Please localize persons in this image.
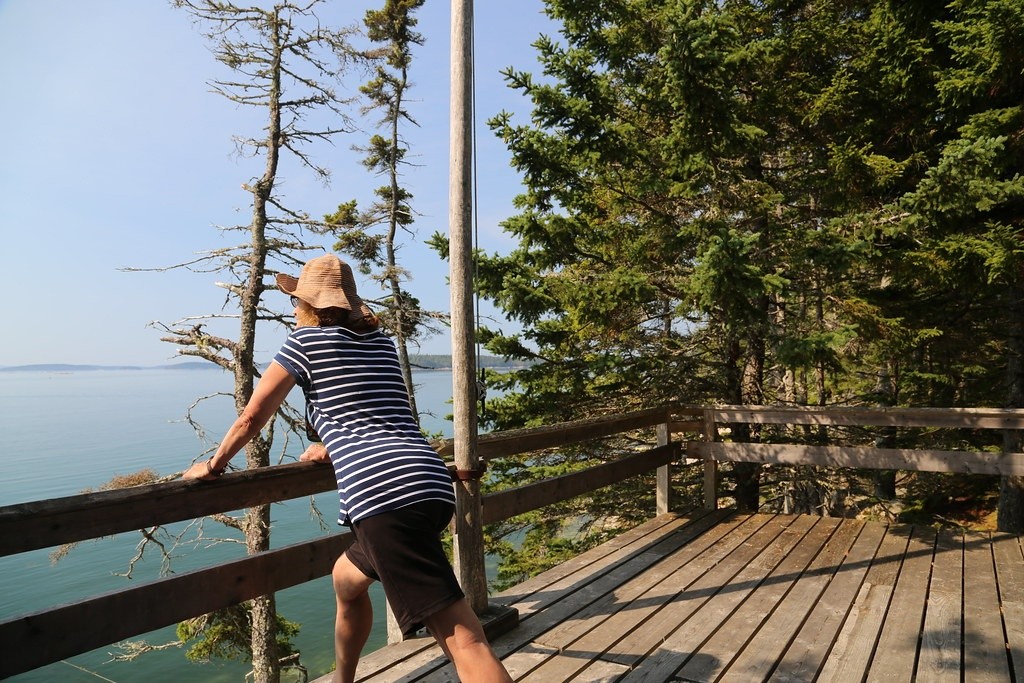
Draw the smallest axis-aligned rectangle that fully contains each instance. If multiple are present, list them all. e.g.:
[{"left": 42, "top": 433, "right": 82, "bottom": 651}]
[{"left": 178, "top": 254, "right": 515, "bottom": 683}]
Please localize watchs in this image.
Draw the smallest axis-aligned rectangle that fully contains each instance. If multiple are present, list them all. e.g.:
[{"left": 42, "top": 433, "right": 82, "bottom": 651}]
[{"left": 207, "top": 455, "right": 228, "bottom": 476}]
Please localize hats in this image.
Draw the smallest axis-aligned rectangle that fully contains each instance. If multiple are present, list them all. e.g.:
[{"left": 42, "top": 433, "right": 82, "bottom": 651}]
[{"left": 274, "top": 255, "right": 376, "bottom": 323}]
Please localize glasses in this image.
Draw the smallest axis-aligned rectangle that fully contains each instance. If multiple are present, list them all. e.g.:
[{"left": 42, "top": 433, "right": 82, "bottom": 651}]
[{"left": 288, "top": 293, "right": 301, "bottom": 307}]
